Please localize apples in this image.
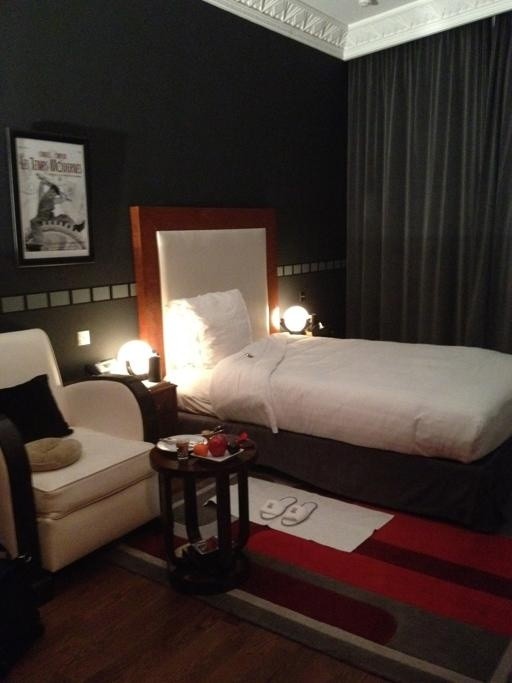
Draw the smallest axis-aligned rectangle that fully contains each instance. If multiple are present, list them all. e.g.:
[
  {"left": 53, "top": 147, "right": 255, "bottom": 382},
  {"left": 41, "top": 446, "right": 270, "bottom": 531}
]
[{"left": 208, "top": 437, "right": 227, "bottom": 457}]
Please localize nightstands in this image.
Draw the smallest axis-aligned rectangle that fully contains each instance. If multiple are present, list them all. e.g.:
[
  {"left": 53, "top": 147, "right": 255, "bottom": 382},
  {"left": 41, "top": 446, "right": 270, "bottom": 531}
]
[{"left": 134, "top": 378, "right": 178, "bottom": 442}]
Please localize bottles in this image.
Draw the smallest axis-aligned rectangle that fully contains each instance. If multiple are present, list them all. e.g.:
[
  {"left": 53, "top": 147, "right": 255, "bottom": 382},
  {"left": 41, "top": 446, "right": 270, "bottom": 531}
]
[{"left": 148, "top": 349, "right": 161, "bottom": 382}]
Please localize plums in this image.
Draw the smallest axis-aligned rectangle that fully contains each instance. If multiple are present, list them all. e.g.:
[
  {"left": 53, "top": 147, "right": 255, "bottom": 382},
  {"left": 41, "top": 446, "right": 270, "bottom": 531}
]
[{"left": 227, "top": 441, "right": 240, "bottom": 454}]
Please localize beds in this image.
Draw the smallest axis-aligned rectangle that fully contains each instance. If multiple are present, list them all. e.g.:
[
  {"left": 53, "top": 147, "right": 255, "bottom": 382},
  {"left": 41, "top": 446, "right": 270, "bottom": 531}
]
[{"left": 131, "top": 205, "right": 512, "bottom": 524}]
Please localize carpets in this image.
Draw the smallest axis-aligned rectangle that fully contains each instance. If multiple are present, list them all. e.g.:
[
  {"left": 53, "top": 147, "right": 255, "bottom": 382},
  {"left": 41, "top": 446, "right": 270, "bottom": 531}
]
[{"left": 104, "top": 466, "right": 512, "bottom": 683}]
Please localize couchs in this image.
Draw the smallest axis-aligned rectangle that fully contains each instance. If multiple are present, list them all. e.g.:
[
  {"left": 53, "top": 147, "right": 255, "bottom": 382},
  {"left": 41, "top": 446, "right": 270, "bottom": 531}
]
[{"left": 0, "top": 327, "right": 159, "bottom": 606}]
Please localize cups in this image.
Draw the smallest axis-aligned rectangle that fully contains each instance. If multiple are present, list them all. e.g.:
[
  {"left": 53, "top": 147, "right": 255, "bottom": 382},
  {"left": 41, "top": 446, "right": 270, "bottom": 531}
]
[{"left": 176, "top": 438, "right": 189, "bottom": 460}]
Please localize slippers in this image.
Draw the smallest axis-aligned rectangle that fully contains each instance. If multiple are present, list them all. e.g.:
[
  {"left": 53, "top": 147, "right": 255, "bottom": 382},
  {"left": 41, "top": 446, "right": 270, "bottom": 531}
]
[
  {"left": 260, "top": 492, "right": 298, "bottom": 519},
  {"left": 280, "top": 501, "right": 319, "bottom": 529}
]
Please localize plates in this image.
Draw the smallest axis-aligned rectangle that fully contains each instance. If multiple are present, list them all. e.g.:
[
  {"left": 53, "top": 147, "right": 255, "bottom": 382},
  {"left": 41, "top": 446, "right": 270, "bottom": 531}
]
[
  {"left": 190, "top": 448, "right": 245, "bottom": 462},
  {"left": 156, "top": 434, "right": 209, "bottom": 453}
]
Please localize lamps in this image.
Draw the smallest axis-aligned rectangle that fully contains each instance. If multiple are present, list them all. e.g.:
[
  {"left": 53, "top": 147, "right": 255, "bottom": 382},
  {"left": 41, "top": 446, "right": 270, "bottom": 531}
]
[
  {"left": 117, "top": 339, "right": 158, "bottom": 381},
  {"left": 279, "top": 304, "right": 311, "bottom": 333}
]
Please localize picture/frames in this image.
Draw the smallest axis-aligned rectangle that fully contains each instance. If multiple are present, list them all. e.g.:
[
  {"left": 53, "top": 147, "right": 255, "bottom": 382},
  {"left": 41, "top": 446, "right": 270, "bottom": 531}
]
[{"left": 5, "top": 125, "right": 96, "bottom": 268}]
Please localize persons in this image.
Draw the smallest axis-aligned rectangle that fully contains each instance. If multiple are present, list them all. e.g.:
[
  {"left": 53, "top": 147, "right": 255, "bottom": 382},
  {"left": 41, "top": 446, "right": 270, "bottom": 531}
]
[{"left": 29, "top": 177, "right": 85, "bottom": 245}]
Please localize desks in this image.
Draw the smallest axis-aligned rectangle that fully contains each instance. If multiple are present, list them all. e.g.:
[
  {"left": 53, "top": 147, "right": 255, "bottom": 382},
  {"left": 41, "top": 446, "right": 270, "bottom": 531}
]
[{"left": 149, "top": 431, "right": 258, "bottom": 564}]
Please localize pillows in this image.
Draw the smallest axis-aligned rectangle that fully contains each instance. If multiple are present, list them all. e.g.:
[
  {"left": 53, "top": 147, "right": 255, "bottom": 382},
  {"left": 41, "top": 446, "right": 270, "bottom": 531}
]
[
  {"left": 165, "top": 288, "right": 251, "bottom": 369},
  {"left": 1, "top": 373, "right": 74, "bottom": 449}
]
